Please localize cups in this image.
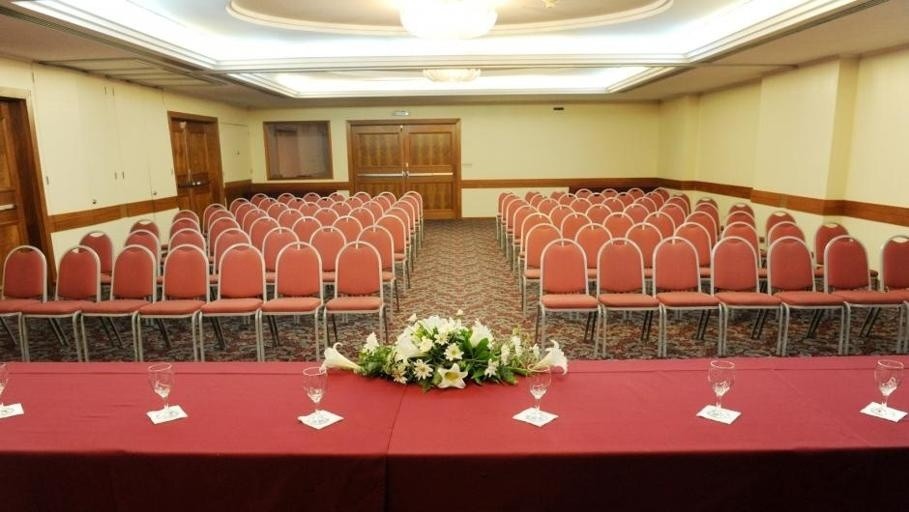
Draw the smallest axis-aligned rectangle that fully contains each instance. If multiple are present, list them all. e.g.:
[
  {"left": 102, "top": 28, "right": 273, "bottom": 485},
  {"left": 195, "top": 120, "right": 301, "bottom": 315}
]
[
  {"left": 0, "top": 361, "right": 15, "bottom": 417},
  {"left": 303, "top": 366, "right": 329, "bottom": 425},
  {"left": 525, "top": 364, "right": 551, "bottom": 421},
  {"left": 147, "top": 362, "right": 179, "bottom": 419},
  {"left": 871, "top": 359, "right": 904, "bottom": 414},
  {"left": 706, "top": 359, "right": 736, "bottom": 417}
]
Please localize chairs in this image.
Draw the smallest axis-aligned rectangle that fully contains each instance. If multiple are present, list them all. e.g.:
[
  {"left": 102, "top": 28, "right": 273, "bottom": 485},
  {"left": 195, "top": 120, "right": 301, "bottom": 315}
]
[
  {"left": 403, "top": 190, "right": 425, "bottom": 244},
  {"left": 700, "top": 237, "right": 782, "bottom": 357},
  {"left": 628, "top": 187, "right": 645, "bottom": 201},
  {"left": 309, "top": 225, "right": 345, "bottom": 289},
  {"left": 300, "top": 202, "right": 320, "bottom": 220},
  {"left": 614, "top": 192, "right": 634, "bottom": 207},
  {"left": 530, "top": 194, "right": 548, "bottom": 206},
  {"left": 512, "top": 206, "right": 537, "bottom": 272},
  {"left": 665, "top": 197, "right": 689, "bottom": 216},
  {"left": 398, "top": 195, "right": 420, "bottom": 260},
  {"left": 356, "top": 225, "right": 400, "bottom": 325},
  {"left": 653, "top": 188, "right": 669, "bottom": 203},
  {"left": 518, "top": 212, "right": 553, "bottom": 294},
  {"left": 602, "top": 188, "right": 618, "bottom": 199},
  {"left": 866, "top": 233, "right": 909, "bottom": 351},
  {"left": 759, "top": 236, "right": 844, "bottom": 357},
  {"left": 207, "top": 218, "right": 241, "bottom": 275},
  {"left": 242, "top": 209, "right": 269, "bottom": 237},
  {"left": 303, "top": 191, "right": 321, "bottom": 206},
  {"left": 671, "top": 192, "right": 691, "bottom": 214},
  {"left": 586, "top": 204, "right": 612, "bottom": 228},
  {"left": 0, "top": 244, "right": 63, "bottom": 365},
  {"left": 659, "top": 203, "right": 685, "bottom": 228},
  {"left": 53, "top": 230, "right": 112, "bottom": 302},
  {"left": 127, "top": 218, "right": 159, "bottom": 243},
  {"left": 362, "top": 200, "right": 382, "bottom": 221},
  {"left": 550, "top": 190, "right": 566, "bottom": 200},
  {"left": 622, "top": 204, "right": 651, "bottom": 224},
  {"left": 624, "top": 221, "right": 666, "bottom": 294},
  {"left": 587, "top": 192, "right": 606, "bottom": 207},
  {"left": 646, "top": 236, "right": 722, "bottom": 358},
  {"left": 286, "top": 197, "right": 308, "bottom": 215},
  {"left": 230, "top": 197, "right": 249, "bottom": 217},
  {"left": 372, "top": 195, "right": 390, "bottom": 213},
  {"left": 315, "top": 208, "right": 339, "bottom": 233},
  {"left": 642, "top": 210, "right": 675, "bottom": 245},
  {"left": 560, "top": 212, "right": 592, "bottom": 245},
  {"left": 721, "top": 222, "right": 768, "bottom": 293},
  {"left": 329, "top": 192, "right": 344, "bottom": 206},
  {"left": 525, "top": 191, "right": 541, "bottom": 203},
  {"left": 330, "top": 216, "right": 363, "bottom": 243},
  {"left": 330, "top": 201, "right": 351, "bottom": 222},
  {"left": 575, "top": 223, "right": 614, "bottom": 281},
  {"left": 814, "top": 221, "right": 878, "bottom": 290},
  {"left": 685, "top": 211, "right": 718, "bottom": 249},
  {"left": 603, "top": 211, "right": 634, "bottom": 245},
  {"left": 266, "top": 202, "right": 290, "bottom": 221},
  {"left": 501, "top": 194, "right": 521, "bottom": 252},
  {"left": 600, "top": 197, "right": 625, "bottom": 217},
  {"left": 724, "top": 211, "right": 757, "bottom": 232},
  {"left": 768, "top": 221, "right": 823, "bottom": 290},
  {"left": 344, "top": 196, "right": 362, "bottom": 211},
  {"left": 506, "top": 199, "right": 529, "bottom": 262},
  {"left": 383, "top": 207, "right": 411, "bottom": 293},
  {"left": 570, "top": 197, "right": 592, "bottom": 214},
  {"left": 101, "top": 229, "right": 160, "bottom": 327},
  {"left": 558, "top": 193, "right": 576, "bottom": 204},
  {"left": 261, "top": 228, "right": 300, "bottom": 292},
  {"left": 591, "top": 237, "right": 663, "bottom": 360},
  {"left": 347, "top": 207, "right": 375, "bottom": 231},
  {"left": 200, "top": 243, "right": 261, "bottom": 361},
  {"left": 379, "top": 190, "right": 396, "bottom": 205},
  {"left": 293, "top": 217, "right": 322, "bottom": 248},
  {"left": 353, "top": 191, "right": 371, "bottom": 207},
  {"left": 576, "top": 187, "right": 592, "bottom": 199},
  {"left": 278, "top": 207, "right": 304, "bottom": 232},
  {"left": 644, "top": 191, "right": 664, "bottom": 212},
  {"left": 376, "top": 216, "right": 410, "bottom": 298},
  {"left": 728, "top": 202, "right": 755, "bottom": 217},
  {"left": 549, "top": 204, "right": 578, "bottom": 229},
  {"left": 210, "top": 227, "right": 252, "bottom": 326},
  {"left": 277, "top": 193, "right": 295, "bottom": 205},
  {"left": 537, "top": 198, "right": 561, "bottom": 217},
  {"left": 201, "top": 203, "right": 226, "bottom": 236},
  {"left": 260, "top": 242, "right": 331, "bottom": 362},
  {"left": 249, "top": 217, "right": 279, "bottom": 251},
  {"left": 762, "top": 211, "right": 796, "bottom": 267},
  {"left": 391, "top": 201, "right": 416, "bottom": 271},
  {"left": 671, "top": 221, "right": 713, "bottom": 286},
  {"left": 696, "top": 197, "right": 718, "bottom": 209},
  {"left": 522, "top": 223, "right": 565, "bottom": 314},
  {"left": 82, "top": 245, "right": 169, "bottom": 363},
  {"left": 157, "top": 227, "right": 210, "bottom": 300},
  {"left": 236, "top": 202, "right": 257, "bottom": 230},
  {"left": 249, "top": 193, "right": 270, "bottom": 209},
  {"left": 161, "top": 209, "right": 199, "bottom": 245},
  {"left": 136, "top": 244, "right": 223, "bottom": 363},
  {"left": 161, "top": 219, "right": 198, "bottom": 266},
  {"left": 633, "top": 197, "right": 657, "bottom": 214},
  {"left": 258, "top": 197, "right": 277, "bottom": 213},
  {"left": 316, "top": 196, "right": 334, "bottom": 213},
  {"left": 811, "top": 235, "right": 904, "bottom": 356},
  {"left": 23, "top": 245, "right": 113, "bottom": 364},
  {"left": 695, "top": 203, "right": 719, "bottom": 239},
  {"left": 324, "top": 241, "right": 389, "bottom": 356},
  {"left": 497, "top": 192, "right": 515, "bottom": 240},
  {"left": 533, "top": 237, "right": 601, "bottom": 358},
  {"left": 206, "top": 208, "right": 235, "bottom": 254}
]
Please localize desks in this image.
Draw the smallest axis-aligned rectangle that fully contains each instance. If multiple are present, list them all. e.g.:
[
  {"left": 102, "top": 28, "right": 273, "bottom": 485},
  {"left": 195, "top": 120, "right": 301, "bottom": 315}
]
[
  {"left": 0, "top": 362, "right": 404, "bottom": 512},
  {"left": 387, "top": 357, "right": 909, "bottom": 512}
]
